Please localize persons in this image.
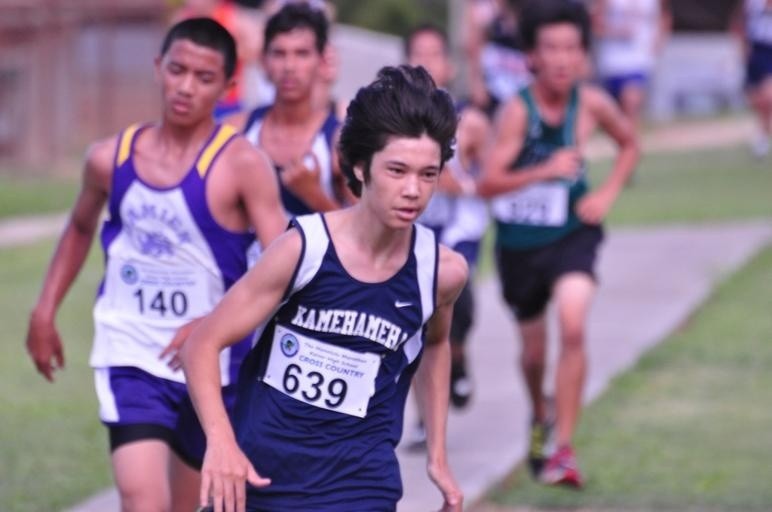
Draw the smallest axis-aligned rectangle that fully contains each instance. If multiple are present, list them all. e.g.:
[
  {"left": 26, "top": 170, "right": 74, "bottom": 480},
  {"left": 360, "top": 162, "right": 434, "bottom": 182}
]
[
  {"left": 394, "top": 22, "right": 491, "bottom": 455},
  {"left": 177, "top": 62, "right": 470, "bottom": 512},
  {"left": 255, "top": 0, "right": 340, "bottom": 118},
  {"left": 727, "top": 0, "right": 772, "bottom": 158},
  {"left": 466, "top": 1, "right": 535, "bottom": 119},
  {"left": 161, "top": 2, "right": 262, "bottom": 134},
  {"left": 477, "top": 1, "right": 644, "bottom": 488},
  {"left": 23, "top": 17, "right": 289, "bottom": 512},
  {"left": 586, "top": 1, "right": 672, "bottom": 184},
  {"left": 219, "top": 4, "right": 357, "bottom": 344}
]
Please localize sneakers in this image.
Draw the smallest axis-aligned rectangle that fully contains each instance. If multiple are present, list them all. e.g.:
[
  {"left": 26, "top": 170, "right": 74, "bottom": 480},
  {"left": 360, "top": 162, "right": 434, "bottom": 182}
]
[
  {"left": 448, "top": 370, "right": 470, "bottom": 406},
  {"left": 529, "top": 421, "right": 583, "bottom": 486}
]
